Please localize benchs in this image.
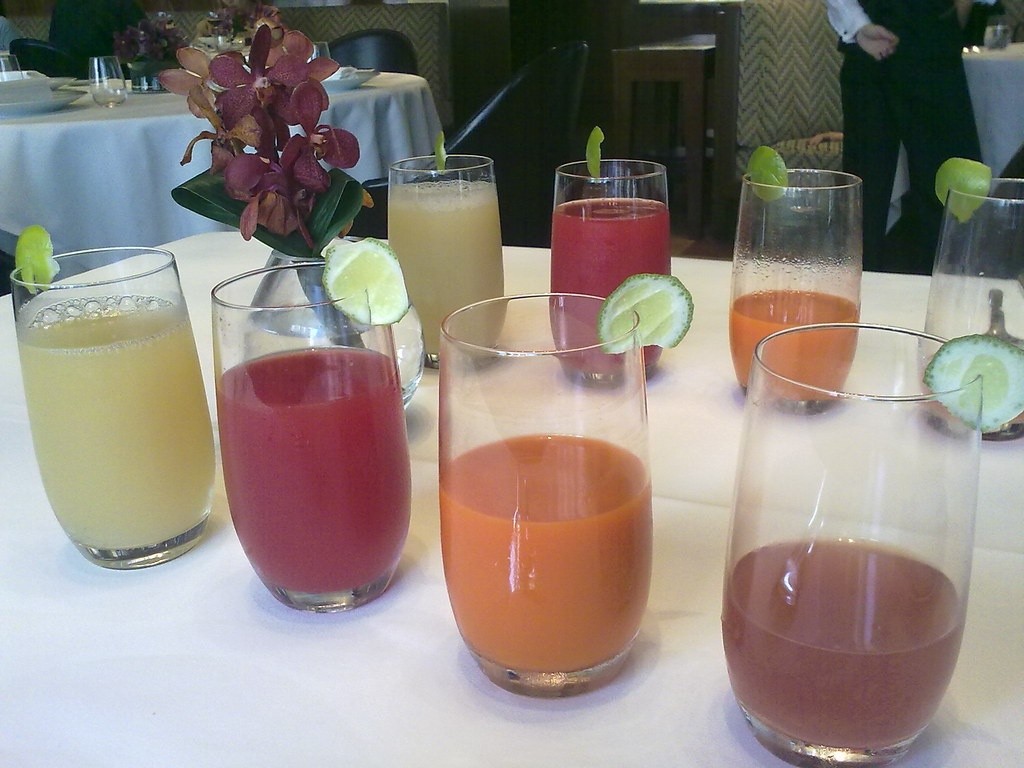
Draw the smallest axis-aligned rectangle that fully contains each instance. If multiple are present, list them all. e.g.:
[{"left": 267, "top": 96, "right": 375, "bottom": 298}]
[{"left": 715, "top": 0, "right": 910, "bottom": 235}]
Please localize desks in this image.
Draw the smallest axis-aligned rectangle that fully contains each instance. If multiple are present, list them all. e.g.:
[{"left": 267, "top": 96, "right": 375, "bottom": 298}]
[
  {"left": 610, "top": 32, "right": 719, "bottom": 240},
  {"left": 962, "top": 41, "right": 1024, "bottom": 197}
]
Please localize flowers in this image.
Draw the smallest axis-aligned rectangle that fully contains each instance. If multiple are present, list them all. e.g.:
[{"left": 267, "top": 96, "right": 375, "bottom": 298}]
[
  {"left": 113, "top": 10, "right": 189, "bottom": 65},
  {"left": 158, "top": 0, "right": 373, "bottom": 347}
]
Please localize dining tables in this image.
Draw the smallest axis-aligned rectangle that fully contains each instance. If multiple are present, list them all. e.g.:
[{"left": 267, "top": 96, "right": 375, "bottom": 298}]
[
  {"left": 0, "top": 232, "right": 1024, "bottom": 768},
  {"left": 0, "top": 68, "right": 449, "bottom": 279}
]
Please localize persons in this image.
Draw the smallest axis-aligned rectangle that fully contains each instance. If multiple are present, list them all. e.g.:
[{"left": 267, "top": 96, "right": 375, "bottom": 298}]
[{"left": 819, "top": 0, "right": 980, "bottom": 276}]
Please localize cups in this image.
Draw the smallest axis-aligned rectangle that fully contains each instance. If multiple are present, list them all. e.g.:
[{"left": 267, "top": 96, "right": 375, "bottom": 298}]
[
  {"left": 721, "top": 321, "right": 983, "bottom": 768},
  {"left": 88, "top": 56, "right": 127, "bottom": 107},
  {"left": 917, "top": 179, "right": 1024, "bottom": 444},
  {"left": 10, "top": 246, "right": 216, "bottom": 569},
  {"left": 0, "top": 54, "right": 23, "bottom": 83},
  {"left": 549, "top": 159, "right": 673, "bottom": 386},
  {"left": 306, "top": 41, "right": 330, "bottom": 63},
  {"left": 387, "top": 155, "right": 505, "bottom": 369},
  {"left": 727, "top": 170, "right": 864, "bottom": 414},
  {"left": 983, "top": 16, "right": 1010, "bottom": 50},
  {"left": 437, "top": 292, "right": 653, "bottom": 697},
  {"left": 210, "top": 262, "right": 413, "bottom": 614}
]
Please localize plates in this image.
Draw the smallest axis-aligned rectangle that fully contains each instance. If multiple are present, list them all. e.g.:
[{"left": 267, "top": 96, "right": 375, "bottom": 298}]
[
  {"left": 320, "top": 72, "right": 381, "bottom": 90},
  {"left": 42, "top": 76, "right": 79, "bottom": 91},
  {"left": 0, "top": 95, "right": 84, "bottom": 117}
]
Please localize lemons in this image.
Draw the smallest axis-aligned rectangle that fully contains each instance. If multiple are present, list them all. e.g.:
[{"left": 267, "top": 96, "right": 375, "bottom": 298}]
[
  {"left": 434, "top": 130, "right": 447, "bottom": 172},
  {"left": 748, "top": 146, "right": 789, "bottom": 201},
  {"left": 323, "top": 237, "right": 408, "bottom": 324},
  {"left": 598, "top": 272, "right": 695, "bottom": 354},
  {"left": 585, "top": 125, "right": 605, "bottom": 179},
  {"left": 923, "top": 333, "right": 1024, "bottom": 432},
  {"left": 935, "top": 157, "right": 991, "bottom": 222},
  {"left": 16, "top": 225, "right": 60, "bottom": 295}
]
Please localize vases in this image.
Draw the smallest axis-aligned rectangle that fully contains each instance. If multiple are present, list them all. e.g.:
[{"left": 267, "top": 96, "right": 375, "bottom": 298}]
[
  {"left": 243, "top": 247, "right": 422, "bottom": 415},
  {"left": 130, "top": 61, "right": 180, "bottom": 94}
]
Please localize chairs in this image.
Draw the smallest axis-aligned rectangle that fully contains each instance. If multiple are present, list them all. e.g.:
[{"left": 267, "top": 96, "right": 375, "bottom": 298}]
[
  {"left": 351, "top": 42, "right": 589, "bottom": 247},
  {"left": 328, "top": 28, "right": 417, "bottom": 76},
  {"left": 9, "top": 38, "right": 81, "bottom": 78}
]
